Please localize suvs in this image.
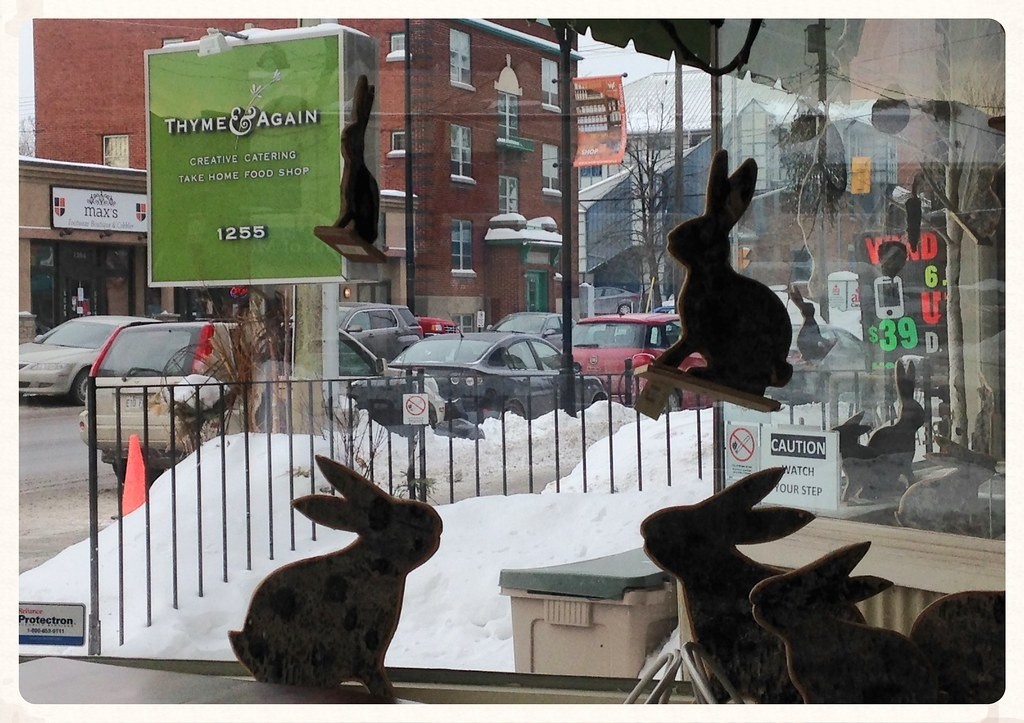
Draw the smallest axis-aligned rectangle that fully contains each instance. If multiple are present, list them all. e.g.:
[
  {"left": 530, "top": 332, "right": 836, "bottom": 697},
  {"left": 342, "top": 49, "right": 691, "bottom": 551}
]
[
  {"left": 413, "top": 315, "right": 456, "bottom": 339},
  {"left": 79, "top": 318, "right": 271, "bottom": 487},
  {"left": 286, "top": 300, "right": 422, "bottom": 363}
]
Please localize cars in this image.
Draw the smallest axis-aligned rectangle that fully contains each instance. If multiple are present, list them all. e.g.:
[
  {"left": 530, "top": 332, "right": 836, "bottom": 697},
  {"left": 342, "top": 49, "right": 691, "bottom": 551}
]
[
  {"left": 486, "top": 311, "right": 578, "bottom": 352},
  {"left": 558, "top": 313, "right": 716, "bottom": 413},
  {"left": 19, "top": 316, "right": 159, "bottom": 406},
  {"left": 764, "top": 282, "right": 864, "bottom": 405},
  {"left": 649, "top": 305, "right": 682, "bottom": 345},
  {"left": 593, "top": 286, "right": 640, "bottom": 316},
  {"left": 387, "top": 332, "right": 609, "bottom": 421}
]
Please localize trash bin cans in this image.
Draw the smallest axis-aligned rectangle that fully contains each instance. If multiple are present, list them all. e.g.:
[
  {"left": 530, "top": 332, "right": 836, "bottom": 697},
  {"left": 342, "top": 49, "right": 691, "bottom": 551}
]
[{"left": 498, "top": 543, "right": 677, "bottom": 680}]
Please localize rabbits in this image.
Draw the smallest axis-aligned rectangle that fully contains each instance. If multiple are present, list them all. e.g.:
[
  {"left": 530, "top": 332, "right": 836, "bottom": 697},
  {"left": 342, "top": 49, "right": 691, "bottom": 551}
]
[
  {"left": 638, "top": 467, "right": 1005, "bottom": 705},
  {"left": 787, "top": 284, "right": 830, "bottom": 364},
  {"left": 225, "top": 455, "right": 444, "bottom": 704},
  {"left": 653, "top": 149, "right": 794, "bottom": 400},
  {"left": 894, "top": 435, "right": 998, "bottom": 539},
  {"left": 332, "top": 73, "right": 381, "bottom": 246},
  {"left": 832, "top": 360, "right": 926, "bottom": 501}
]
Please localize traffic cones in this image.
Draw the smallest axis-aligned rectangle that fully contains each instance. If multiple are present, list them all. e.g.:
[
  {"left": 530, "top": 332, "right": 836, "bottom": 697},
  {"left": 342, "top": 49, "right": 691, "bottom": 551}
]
[{"left": 110, "top": 432, "right": 146, "bottom": 519}]
